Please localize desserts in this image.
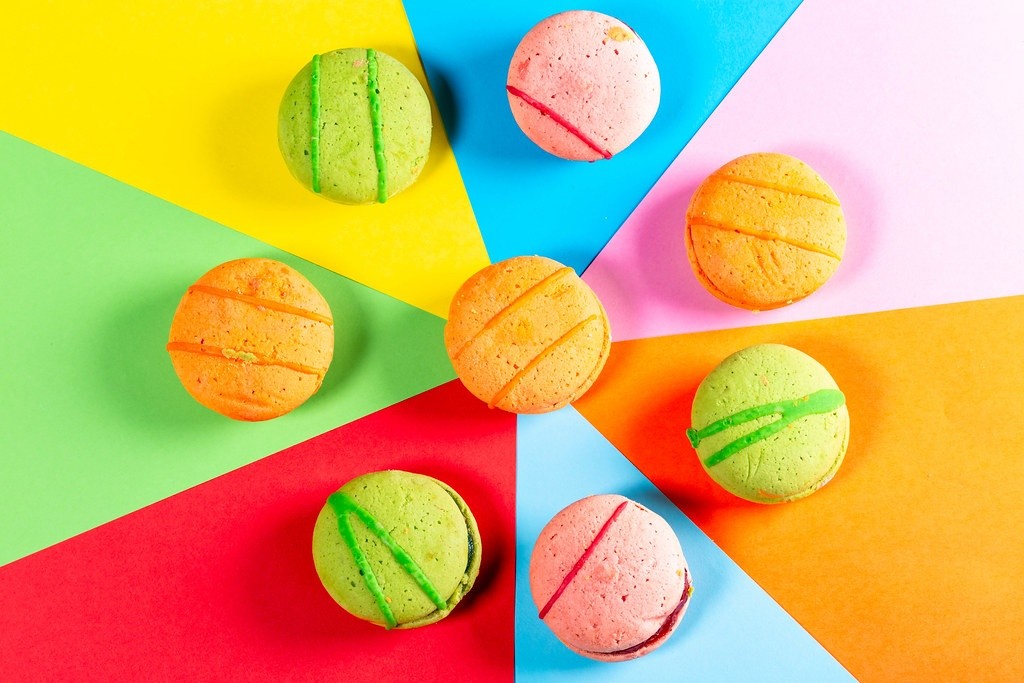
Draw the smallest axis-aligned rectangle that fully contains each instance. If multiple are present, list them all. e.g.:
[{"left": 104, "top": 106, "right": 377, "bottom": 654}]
[{"left": 167, "top": 8, "right": 852, "bottom": 657}]
